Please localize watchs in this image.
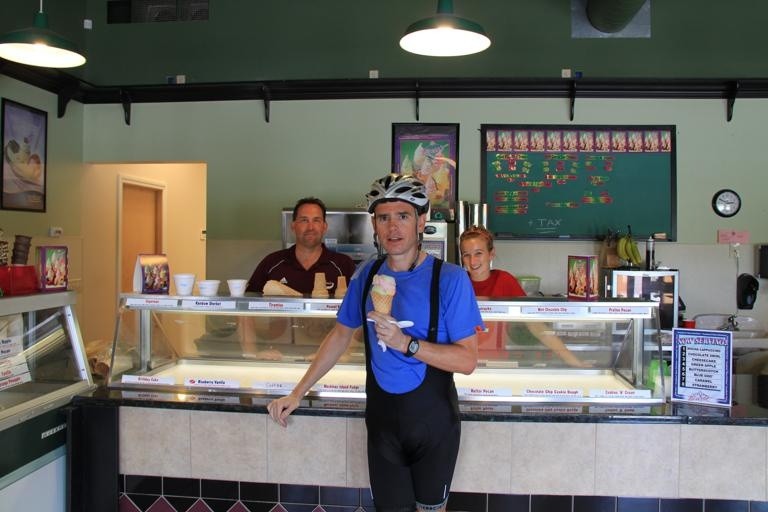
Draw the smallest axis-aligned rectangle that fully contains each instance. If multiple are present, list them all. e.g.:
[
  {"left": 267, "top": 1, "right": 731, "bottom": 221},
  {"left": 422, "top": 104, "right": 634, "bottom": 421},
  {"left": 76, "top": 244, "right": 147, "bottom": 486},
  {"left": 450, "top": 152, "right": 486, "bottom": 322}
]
[{"left": 403, "top": 335, "right": 420, "bottom": 357}]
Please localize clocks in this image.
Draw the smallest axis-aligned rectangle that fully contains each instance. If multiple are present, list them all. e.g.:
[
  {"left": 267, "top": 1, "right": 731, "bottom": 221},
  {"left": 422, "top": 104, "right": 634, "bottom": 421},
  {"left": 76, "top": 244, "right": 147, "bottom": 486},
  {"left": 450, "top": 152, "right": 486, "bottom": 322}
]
[{"left": 712, "top": 189, "right": 741, "bottom": 217}]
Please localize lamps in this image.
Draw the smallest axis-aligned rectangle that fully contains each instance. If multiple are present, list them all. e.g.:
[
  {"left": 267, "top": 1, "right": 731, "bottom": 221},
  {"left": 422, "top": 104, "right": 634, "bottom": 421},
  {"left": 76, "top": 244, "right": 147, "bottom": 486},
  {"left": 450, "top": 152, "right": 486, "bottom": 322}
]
[
  {"left": 400, "top": 1, "right": 492, "bottom": 58},
  {"left": 0, "top": 0, "right": 87, "bottom": 68}
]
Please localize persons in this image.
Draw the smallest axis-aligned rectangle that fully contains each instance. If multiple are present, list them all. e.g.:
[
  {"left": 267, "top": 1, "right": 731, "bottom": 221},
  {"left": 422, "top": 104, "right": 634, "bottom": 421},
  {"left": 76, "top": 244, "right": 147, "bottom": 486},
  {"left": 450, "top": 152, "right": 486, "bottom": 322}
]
[
  {"left": 460, "top": 225, "right": 593, "bottom": 368},
  {"left": 267, "top": 172, "right": 486, "bottom": 512},
  {"left": 236, "top": 197, "right": 361, "bottom": 362}
]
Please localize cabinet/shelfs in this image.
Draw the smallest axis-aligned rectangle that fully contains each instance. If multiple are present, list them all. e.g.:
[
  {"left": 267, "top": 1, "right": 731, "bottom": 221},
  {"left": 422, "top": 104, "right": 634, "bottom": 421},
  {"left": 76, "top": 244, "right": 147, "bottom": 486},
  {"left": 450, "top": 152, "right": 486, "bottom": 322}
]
[
  {"left": 0, "top": 291, "right": 94, "bottom": 420},
  {"left": 104, "top": 293, "right": 671, "bottom": 404}
]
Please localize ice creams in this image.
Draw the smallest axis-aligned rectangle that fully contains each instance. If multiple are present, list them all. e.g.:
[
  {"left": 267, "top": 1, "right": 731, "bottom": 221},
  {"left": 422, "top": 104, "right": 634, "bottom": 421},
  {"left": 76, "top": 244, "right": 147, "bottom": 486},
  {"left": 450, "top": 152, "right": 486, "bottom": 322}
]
[{"left": 370, "top": 274, "right": 397, "bottom": 315}]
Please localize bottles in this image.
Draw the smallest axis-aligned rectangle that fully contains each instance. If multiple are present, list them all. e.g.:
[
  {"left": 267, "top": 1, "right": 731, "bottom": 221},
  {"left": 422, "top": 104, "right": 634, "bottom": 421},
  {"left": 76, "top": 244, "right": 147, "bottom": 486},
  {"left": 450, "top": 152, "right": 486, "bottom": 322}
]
[{"left": 645, "top": 236, "right": 655, "bottom": 272}]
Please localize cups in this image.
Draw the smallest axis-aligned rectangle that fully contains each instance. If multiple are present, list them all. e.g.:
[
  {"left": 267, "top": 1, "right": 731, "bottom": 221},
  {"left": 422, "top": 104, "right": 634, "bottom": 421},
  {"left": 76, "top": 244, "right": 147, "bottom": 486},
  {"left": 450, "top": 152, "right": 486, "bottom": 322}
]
[
  {"left": 12, "top": 235, "right": 31, "bottom": 279},
  {"left": 174, "top": 273, "right": 193, "bottom": 297},
  {"left": 227, "top": 278, "right": 247, "bottom": 297},
  {"left": 194, "top": 279, "right": 223, "bottom": 298}
]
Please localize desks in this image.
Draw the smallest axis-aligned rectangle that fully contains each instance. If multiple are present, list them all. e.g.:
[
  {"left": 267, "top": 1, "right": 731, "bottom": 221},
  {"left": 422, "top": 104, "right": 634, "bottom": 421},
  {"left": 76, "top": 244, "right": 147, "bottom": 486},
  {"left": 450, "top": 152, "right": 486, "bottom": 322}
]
[{"left": 91, "top": 366, "right": 154, "bottom": 397}]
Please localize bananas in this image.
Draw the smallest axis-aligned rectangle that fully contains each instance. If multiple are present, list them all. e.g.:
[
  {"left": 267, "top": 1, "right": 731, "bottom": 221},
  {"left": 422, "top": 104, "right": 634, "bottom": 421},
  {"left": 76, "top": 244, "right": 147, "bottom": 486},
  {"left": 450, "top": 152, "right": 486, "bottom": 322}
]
[{"left": 617, "top": 235, "right": 642, "bottom": 267}]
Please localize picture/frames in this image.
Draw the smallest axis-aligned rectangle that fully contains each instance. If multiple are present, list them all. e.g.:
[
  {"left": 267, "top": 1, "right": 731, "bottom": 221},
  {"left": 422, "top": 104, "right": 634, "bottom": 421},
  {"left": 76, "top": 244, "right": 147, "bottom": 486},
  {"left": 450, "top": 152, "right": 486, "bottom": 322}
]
[
  {"left": 392, "top": 123, "right": 460, "bottom": 222},
  {"left": 0, "top": 97, "right": 48, "bottom": 212}
]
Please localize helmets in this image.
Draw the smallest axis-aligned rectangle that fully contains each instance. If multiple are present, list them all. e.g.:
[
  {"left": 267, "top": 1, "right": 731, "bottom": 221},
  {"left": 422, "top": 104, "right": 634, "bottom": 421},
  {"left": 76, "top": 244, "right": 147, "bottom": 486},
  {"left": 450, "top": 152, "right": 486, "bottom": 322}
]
[{"left": 366, "top": 173, "right": 429, "bottom": 216}]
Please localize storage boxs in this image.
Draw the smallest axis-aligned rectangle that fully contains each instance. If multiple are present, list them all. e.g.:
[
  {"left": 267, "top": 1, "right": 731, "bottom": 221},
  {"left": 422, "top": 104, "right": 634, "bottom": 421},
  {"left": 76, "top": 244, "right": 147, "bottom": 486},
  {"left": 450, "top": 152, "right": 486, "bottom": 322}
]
[{"left": 518, "top": 277, "right": 540, "bottom": 296}]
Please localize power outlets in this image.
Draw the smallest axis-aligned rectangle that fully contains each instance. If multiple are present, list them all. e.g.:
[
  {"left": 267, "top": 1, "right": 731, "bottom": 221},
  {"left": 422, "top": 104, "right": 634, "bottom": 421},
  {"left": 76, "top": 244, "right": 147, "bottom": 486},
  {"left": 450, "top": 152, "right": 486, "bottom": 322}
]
[{"left": 50, "top": 225, "right": 63, "bottom": 239}]
